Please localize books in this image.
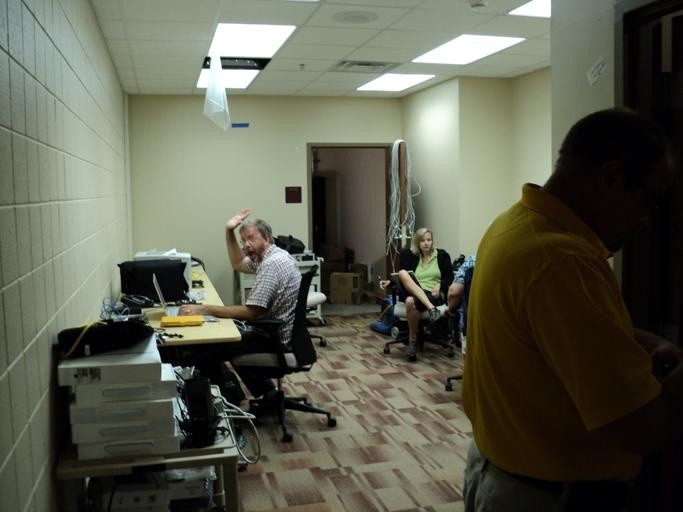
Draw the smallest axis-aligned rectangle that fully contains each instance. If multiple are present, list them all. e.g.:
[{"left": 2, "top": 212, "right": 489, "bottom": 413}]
[
  {"left": 391, "top": 270, "right": 423, "bottom": 288},
  {"left": 160, "top": 314, "right": 204, "bottom": 326}
]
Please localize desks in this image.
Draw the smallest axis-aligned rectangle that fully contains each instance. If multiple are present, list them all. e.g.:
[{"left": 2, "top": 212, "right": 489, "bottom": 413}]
[{"left": 55, "top": 259, "right": 242, "bottom": 512}]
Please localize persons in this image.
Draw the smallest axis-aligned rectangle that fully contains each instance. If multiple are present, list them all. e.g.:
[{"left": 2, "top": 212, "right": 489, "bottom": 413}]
[
  {"left": 377, "top": 228, "right": 454, "bottom": 362},
  {"left": 447, "top": 255, "right": 476, "bottom": 333},
  {"left": 461, "top": 106, "right": 682, "bottom": 512},
  {"left": 177, "top": 207, "right": 303, "bottom": 415}
]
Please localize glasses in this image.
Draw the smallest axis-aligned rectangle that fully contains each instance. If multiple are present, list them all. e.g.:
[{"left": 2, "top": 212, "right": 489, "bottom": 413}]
[{"left": 239, "top": 237, "right": 255, "bottom": 245}]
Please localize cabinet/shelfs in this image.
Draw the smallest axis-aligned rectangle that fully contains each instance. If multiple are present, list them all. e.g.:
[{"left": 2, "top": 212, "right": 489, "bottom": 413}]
[{"left": 235, "top": 255, "right": 325, "bottom": 327}]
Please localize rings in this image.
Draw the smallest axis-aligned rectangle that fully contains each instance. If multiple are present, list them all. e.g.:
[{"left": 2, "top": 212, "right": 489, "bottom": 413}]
[{"left": 187, "top": 310, "right": 191, "bottom": 313}]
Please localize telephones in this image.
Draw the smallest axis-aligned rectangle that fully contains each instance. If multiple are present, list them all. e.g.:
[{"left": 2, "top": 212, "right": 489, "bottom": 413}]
[{"left": 121, "top": 295, "right": 152, "bottom": 308}]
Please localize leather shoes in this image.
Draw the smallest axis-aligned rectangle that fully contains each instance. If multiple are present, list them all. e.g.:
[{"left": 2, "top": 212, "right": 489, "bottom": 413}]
[{"left": 251, "top": 391, "right": 279, "bottom": 408}]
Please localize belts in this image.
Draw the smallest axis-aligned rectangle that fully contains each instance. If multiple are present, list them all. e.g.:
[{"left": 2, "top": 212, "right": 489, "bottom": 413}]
[{"left": 485, "top": 460, "right": 643, "bottom": 492}]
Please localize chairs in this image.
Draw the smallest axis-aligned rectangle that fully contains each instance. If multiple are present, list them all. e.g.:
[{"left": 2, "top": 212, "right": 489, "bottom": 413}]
[
  {"left": 219, "top": 264, "right": 337, "bottom": 443},
  {"left": 384, "top": 250, "right": 456, "bottom": 357}
]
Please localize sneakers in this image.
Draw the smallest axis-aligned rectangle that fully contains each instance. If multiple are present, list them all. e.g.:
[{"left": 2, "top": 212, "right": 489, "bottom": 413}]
[
  {"left": 407, "top": 343, "right": 416, "bottom": 357},
  {"left": 427, "top": 307, "right": 440, "bottom": 323}
]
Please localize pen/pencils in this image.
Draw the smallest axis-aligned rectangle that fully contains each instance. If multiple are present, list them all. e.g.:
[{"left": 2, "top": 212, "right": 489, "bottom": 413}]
[{"left": 378, "top": 274, "right": 383, "bottom": 286}]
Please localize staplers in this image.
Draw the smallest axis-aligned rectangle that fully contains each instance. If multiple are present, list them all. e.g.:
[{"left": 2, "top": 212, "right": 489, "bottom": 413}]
[{"left": 191, "top": 280, "right": 203, "bottom": 288}]
